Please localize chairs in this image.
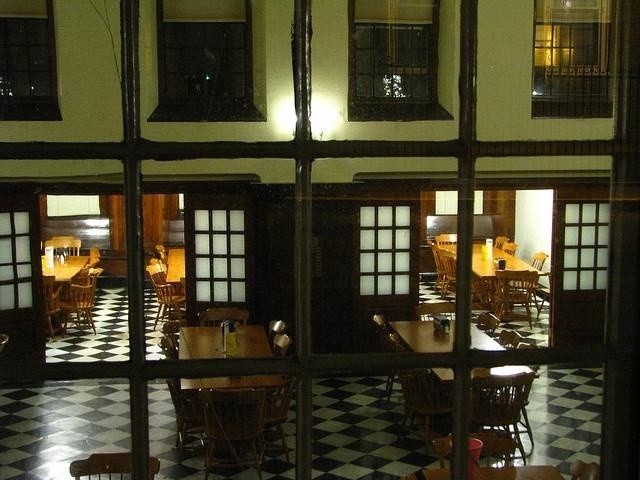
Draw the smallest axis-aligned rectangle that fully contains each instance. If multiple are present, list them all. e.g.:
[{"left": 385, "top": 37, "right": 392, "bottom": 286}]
[
  {"left": 569, "top": 460, "right": 601, "bottom": 480},
  {"left": 71, "top": 454, "right": 160, "bottom": 480},
  {"left": 41, "top": 237, "right": 186, "bottom": 336},
  {"left": 428, "top": 235, "right": 552, "bottom": 330},
  {"left": 160, "top": 308, "right": 298, "bottom": 480},
  {"left": 372, "top": 303, "right": 543, "bottom": 469}
]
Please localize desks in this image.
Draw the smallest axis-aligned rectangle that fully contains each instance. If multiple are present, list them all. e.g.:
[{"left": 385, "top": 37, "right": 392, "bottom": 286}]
[{"left": 422, "top": 464, "right": 567, "bottom": 480}]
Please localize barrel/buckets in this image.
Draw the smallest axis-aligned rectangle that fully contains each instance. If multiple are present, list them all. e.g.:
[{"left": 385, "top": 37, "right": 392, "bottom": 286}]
[{"left": 448, "top": 437, "right": 483, "bottom": 480}]
